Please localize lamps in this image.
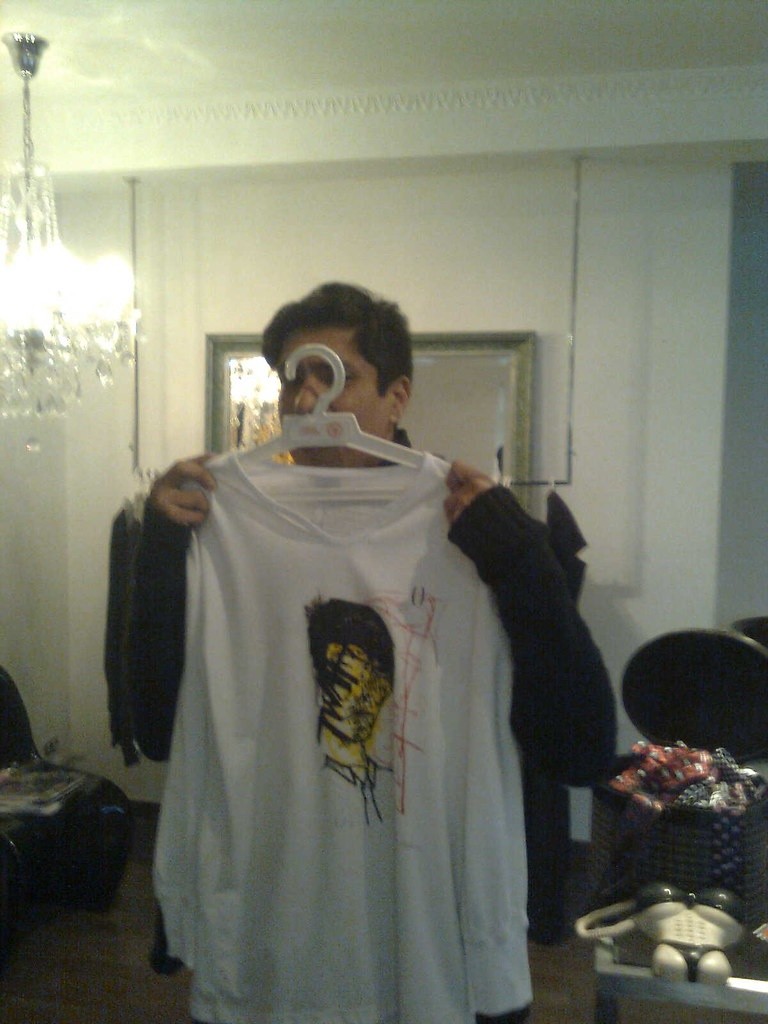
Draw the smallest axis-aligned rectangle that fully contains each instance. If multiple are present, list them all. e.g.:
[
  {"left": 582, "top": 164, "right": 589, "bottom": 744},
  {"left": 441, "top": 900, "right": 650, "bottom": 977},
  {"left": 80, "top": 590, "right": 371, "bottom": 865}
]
[{"left": 0, "top": 31, "right": 151, "bottom": 457}]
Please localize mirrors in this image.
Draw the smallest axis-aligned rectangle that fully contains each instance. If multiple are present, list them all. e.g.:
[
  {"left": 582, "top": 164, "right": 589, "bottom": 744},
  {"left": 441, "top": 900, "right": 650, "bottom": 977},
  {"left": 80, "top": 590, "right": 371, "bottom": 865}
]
[
  {"left": 713, "top": 161, "right": 768, "bottom": 649},
  {"left": 203, "top": 329, "right": 537, "bottom": 513}
]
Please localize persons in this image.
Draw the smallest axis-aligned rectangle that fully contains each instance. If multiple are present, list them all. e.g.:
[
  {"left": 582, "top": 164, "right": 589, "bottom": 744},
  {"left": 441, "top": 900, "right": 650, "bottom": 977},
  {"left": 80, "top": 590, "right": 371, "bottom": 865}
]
[{"left": 105, "top": 284, "right": 618, "bottom": 1024}]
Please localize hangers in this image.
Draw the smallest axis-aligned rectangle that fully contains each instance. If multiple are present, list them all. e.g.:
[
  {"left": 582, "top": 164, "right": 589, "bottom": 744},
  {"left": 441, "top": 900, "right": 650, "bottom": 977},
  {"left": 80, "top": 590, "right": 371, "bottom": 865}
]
[{"left": 238, "top": 342, "right": 422, "bottom": 503}]
